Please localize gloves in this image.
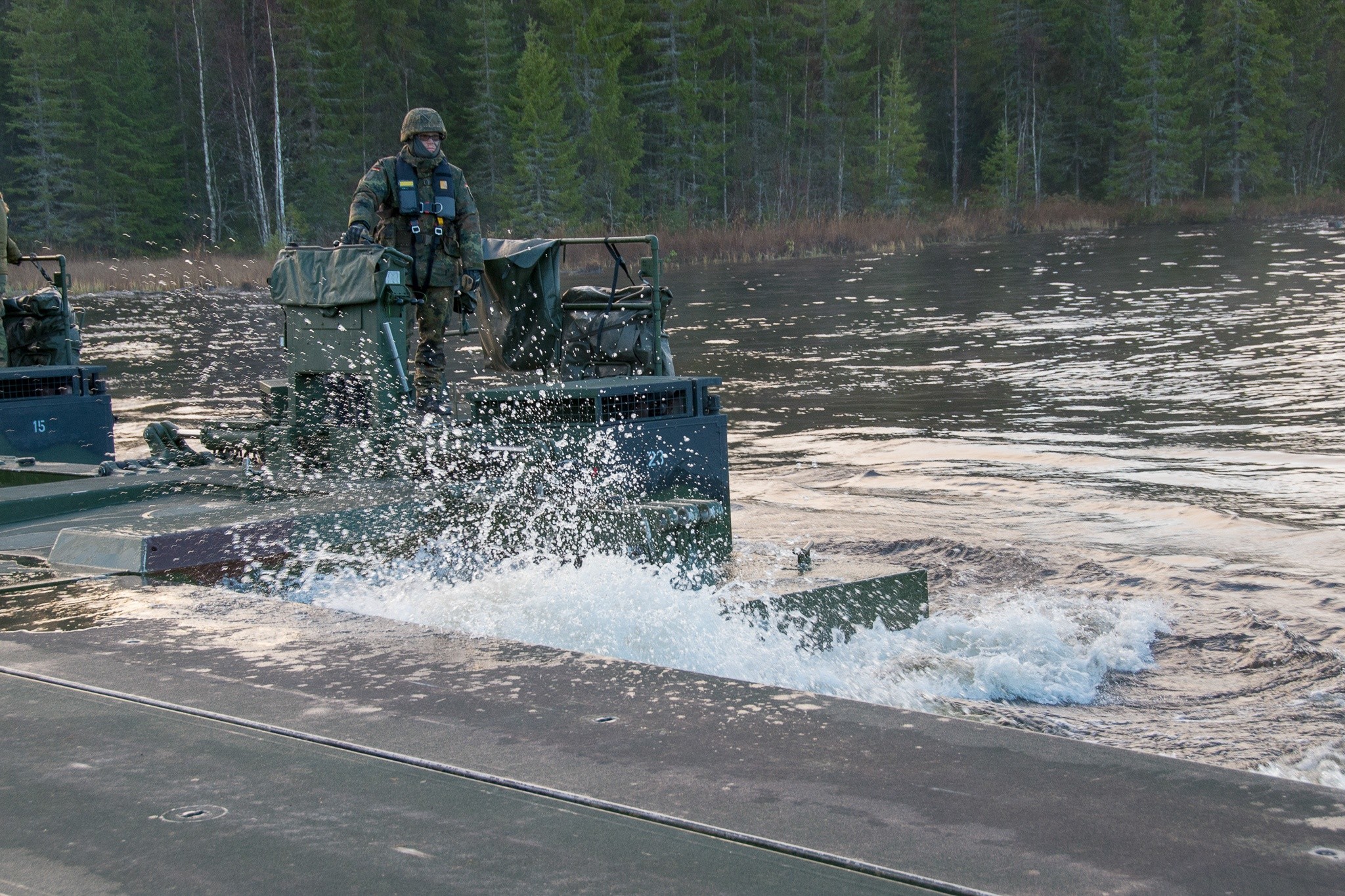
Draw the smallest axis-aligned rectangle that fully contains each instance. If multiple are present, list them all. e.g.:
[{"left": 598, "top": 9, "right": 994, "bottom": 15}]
[
  {"left": 346, "top": 223, "right": 369, "bottom": 244},
  {"left": 461, "top": 270, "right": 481, "bottom": 292}
]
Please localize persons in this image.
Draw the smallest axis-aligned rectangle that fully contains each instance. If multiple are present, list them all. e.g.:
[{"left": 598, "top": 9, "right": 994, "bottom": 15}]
[
  {"left": 0, "top": 193, "right": 21, "bottom": 367},
  {"left": 348, "top": 108, "right": 484, "bottom": 416}
]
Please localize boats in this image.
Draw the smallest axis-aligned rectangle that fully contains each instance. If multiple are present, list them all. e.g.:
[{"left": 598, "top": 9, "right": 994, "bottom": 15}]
[
  {"left": 99, "top": 227, "right": 734, "bottom": 575},
  {"left": 0, "top": 190, "right": 117, "bottom": 477}
]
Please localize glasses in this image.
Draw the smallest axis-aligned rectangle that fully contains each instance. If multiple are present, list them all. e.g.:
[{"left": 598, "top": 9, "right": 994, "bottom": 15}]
[{"left": 418, "top": 134, "right": 440, "bottom": 141}]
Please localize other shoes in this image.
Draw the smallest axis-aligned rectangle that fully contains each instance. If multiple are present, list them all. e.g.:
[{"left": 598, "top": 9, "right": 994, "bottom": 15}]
[{"left": 416, "top": 396, "right": 452, "bottom": 414}]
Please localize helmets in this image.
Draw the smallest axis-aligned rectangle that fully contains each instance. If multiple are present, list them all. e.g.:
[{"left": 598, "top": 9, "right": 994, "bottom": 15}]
[{"left": 401, "top": 108, "right": 447, "bottom": 142}]
